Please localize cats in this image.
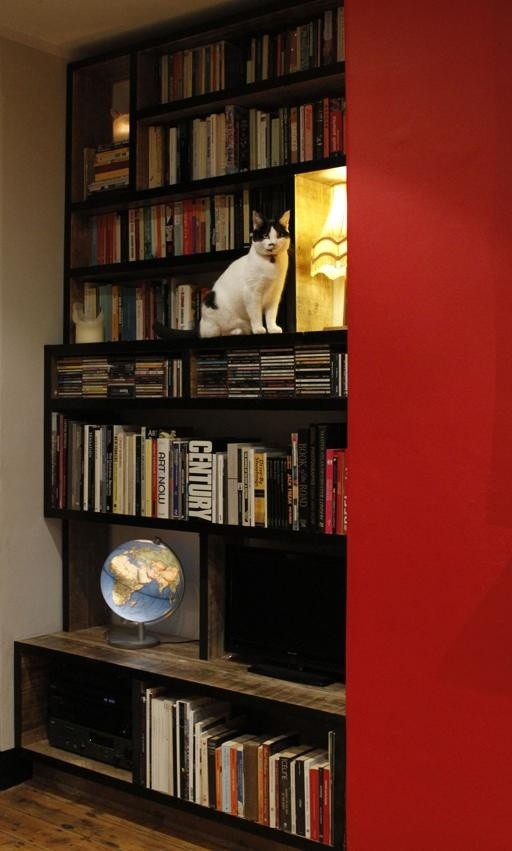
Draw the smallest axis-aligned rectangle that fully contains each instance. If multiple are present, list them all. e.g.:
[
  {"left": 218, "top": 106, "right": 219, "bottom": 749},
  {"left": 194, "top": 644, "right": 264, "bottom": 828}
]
[{"left": 152, "top": 210, "right": 290, "bottom": 339}]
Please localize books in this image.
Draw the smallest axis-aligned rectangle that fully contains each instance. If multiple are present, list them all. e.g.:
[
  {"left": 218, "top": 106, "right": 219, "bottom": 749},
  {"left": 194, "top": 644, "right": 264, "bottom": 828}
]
[
  {"left": 49, "top": 410, "right": 348, "bottom": 534},
  {"left": 148, "top": 98, "right": 346, "bottom": 187},
  {"left": 142, "top": 683, "right": 344, "bottom": 847},
  {"left": 162, "top": 6, "right": 345, "bottom": 106},
  {"left": 89, "top": 188, "right": 290, "bottom": 266},
  {"left": 51, "top": 339, "right": 349, "bottom": 399},
  {"left": 84, "top": 281, "right": 210, "bottom": 342}
]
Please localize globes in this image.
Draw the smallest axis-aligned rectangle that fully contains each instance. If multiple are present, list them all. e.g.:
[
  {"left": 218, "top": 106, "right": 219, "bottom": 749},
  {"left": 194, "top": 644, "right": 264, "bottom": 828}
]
[{"left": 100, "top": 536, "right": 185, "bottom": 650}]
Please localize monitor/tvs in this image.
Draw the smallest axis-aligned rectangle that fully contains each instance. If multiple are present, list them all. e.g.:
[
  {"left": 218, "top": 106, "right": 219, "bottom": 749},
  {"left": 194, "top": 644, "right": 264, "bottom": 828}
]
[{"left": 224, "top": 544, "right": 347, "bottom": 685}]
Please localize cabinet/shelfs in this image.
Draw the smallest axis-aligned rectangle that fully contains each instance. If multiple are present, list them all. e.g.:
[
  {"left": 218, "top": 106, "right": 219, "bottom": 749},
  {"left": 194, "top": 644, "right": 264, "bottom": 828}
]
[{"left": 14, "top": 0, "right": 347, "bottom": 851}]
